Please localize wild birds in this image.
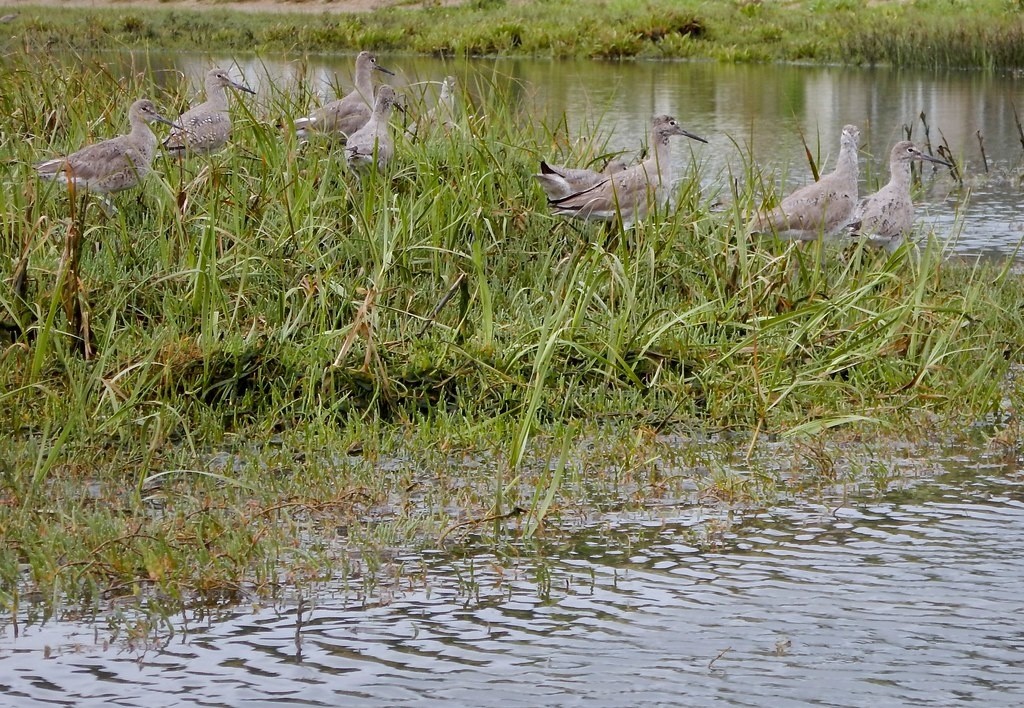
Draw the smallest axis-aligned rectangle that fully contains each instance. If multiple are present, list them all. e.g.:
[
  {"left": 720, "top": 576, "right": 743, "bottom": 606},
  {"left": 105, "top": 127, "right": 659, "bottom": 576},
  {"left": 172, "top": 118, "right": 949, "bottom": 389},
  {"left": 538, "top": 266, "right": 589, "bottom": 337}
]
[
  {"left": 158, "top": 68, "right": 256, "bottom": 159},
  {"left": 532, "top": 115, "right": 709, "bottom": 231},
  {"left": 35, "top": 99, "right": 185, "bottom": 206},
  {"left": 757, "top": 125, "right": 952, "bottom": 258},
  {"left": 275, "top": 50, "right": 455, "bottom": 174}
]
